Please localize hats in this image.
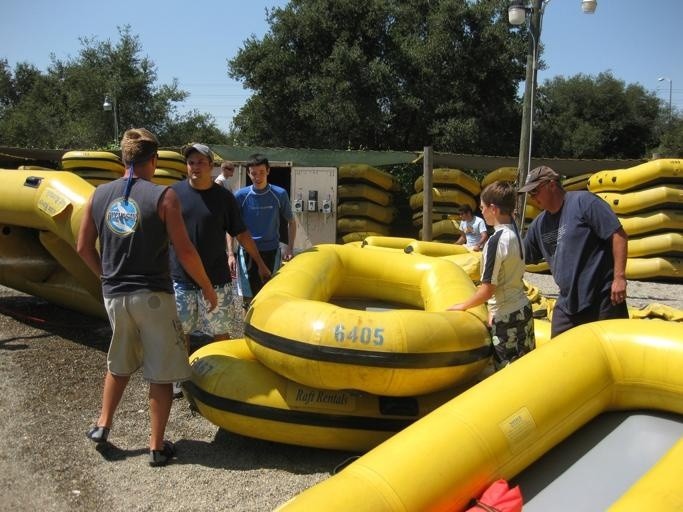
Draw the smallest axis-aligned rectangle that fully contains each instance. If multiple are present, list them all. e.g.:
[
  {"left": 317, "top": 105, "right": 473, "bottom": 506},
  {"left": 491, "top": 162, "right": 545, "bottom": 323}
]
[
  {"left": 518, "top": 164, "right": 559, "bottom": 194},
  {"left": 185, "top": 142, "right": 213, "bottom": 162}
]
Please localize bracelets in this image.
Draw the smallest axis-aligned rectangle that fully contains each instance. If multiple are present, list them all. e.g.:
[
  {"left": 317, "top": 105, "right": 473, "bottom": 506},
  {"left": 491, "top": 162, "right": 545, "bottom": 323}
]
[{"left": 476, "top": 244, "right": 481, "bottom": 247}]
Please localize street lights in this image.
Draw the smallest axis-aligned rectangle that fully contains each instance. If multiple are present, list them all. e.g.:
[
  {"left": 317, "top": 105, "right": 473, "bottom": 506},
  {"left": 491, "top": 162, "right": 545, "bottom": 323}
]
[
  {"left": 503, "top": 0, "right": 596, "bottom": 259},
  {"left": 98, "top": 90, "right": 127, "bottom": 152},
  {"left": 657, "top": 75, "right": 673, "bottom": 152}
]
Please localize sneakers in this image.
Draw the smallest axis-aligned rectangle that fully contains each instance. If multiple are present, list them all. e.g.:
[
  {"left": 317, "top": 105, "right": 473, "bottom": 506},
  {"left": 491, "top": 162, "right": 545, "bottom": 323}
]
[
  {"left": 84, "top": 426, "right": 109, "bottom": 443},
  {"left": 149, "top": 440, "right": 175, "bottom": 466}
]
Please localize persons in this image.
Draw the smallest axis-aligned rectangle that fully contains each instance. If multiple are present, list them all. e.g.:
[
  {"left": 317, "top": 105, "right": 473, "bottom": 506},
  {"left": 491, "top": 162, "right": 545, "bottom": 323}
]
[
  {"left": 226, "top": 153, "right": 297, "bottom": 320},
  {"left": 453, "top": 204, "right": 489, "bottom": 252},
  {"left": 214, "top": 160, "right": 235, "bottom": 194},
  {"left": 445, "top": 181, "right": 537, "bottom": 372},
  {"left": 516, "top": 165, "right": 630, "bottom": 339},
  {"left": 167, "top": 142, "right": 273, "bottom": 399},
  {"left": 85, "top": 128, "right": 218, "bottom": 467}
]
